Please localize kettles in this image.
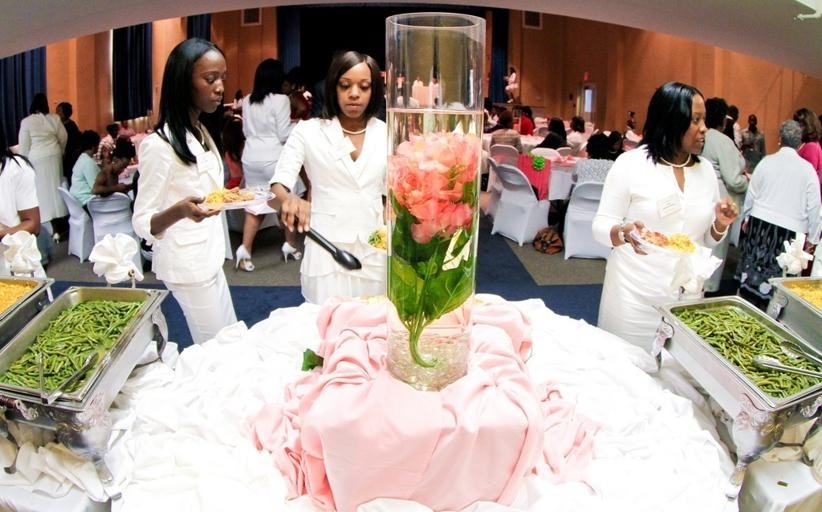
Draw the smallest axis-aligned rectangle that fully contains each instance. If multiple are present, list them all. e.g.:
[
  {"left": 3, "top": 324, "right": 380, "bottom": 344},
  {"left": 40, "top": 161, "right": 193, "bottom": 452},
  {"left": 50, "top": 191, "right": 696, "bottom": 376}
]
[
  {"left": 0, "top": 301, "right": 143, "bottom": 393},
  {"left": 675, "top": 309, "right": 822, "bottom": 398}
]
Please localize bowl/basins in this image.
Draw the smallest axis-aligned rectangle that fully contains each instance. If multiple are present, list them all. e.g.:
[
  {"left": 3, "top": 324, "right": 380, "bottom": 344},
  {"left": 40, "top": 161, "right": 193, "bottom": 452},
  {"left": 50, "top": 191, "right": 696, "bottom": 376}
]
[
  {"left": 194, "top": 125, "right": 204, "bottom": 147},
  {"left": 342, "top": 126, "right": 366, "bottom": 136},
  {"left": 659, "top": 153, "right": 692, "bottom": 169}
]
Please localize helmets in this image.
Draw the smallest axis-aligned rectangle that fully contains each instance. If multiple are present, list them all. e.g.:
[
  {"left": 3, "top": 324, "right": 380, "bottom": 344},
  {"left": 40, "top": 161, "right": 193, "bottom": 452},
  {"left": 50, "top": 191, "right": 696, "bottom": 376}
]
[
  {"left": 627, "top": 227, "right": 697, "bottom": 262},
  {"left": 196, "top": 188, "right": 275, "bottom": 212}
]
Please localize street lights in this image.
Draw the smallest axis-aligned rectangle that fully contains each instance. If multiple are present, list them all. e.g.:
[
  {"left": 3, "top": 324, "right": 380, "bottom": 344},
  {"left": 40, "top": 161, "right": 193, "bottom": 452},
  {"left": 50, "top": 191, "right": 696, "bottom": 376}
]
[{"left": 533, "top": 226, "right": 563, "bottom": 255}]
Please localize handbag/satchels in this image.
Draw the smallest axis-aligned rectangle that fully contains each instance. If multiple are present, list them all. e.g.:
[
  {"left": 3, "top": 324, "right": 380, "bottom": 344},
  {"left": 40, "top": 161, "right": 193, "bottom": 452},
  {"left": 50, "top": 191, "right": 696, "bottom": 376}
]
[{"left": 712, "top": 222, "right": 729, "bottom": 236}]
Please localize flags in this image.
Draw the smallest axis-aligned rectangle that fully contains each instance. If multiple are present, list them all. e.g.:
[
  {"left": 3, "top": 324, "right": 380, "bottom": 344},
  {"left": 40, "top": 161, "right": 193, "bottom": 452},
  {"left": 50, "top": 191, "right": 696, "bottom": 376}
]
[{"left": 383, "top": 8, "right": 492, "bottom": 393}]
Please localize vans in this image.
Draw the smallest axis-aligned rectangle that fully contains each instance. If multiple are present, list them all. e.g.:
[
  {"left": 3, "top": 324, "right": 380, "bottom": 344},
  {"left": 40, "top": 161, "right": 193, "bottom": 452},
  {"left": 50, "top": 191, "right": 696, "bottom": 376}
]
[
  {"left": 236, "top": 245, "right": 255, "bottom": 271},
  {"left": 282, "top": 242, "right": 302, "bottom": 264}
]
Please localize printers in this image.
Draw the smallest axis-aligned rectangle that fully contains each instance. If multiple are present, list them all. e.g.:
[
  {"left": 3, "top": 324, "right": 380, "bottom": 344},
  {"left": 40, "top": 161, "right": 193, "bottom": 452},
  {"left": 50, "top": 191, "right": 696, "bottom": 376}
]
[
  {"left": 34, "top": 351, "right": 101, "bottom": 409},
  {"left": 277, "top": 208, "right": 361, "bottom": 272},
  {"left": 748, "top": 338, "right": 821, "bottom": 383}
]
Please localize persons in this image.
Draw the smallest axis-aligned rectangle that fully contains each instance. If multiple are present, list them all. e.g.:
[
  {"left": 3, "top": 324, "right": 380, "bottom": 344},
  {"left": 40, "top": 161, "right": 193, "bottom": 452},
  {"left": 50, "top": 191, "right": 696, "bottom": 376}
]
[
  {"left": 378, "top": 65, "right": 637, "bottom": 193},
  {"left": 267, "top": 49, "right": 390, "bottom": 305},
  {"left": 200, "top": 56, "right": 322, "bottom": 272},
  {"left": 0, "top": 92, "right": 152, "bottom": 282},
  {"left": 592, "top": 81, "right": 738, "bottom": 356},
  {"left": 700, "top": 97, "right": 822, "bottom": 313},
  {"left": 132, "top": 36, "right": 237, "bottom": 345}
]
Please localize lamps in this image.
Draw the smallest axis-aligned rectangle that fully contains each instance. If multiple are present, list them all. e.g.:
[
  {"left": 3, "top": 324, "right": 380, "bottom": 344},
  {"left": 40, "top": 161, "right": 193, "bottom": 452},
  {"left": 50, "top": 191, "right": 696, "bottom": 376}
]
[
  {"left": 562, "top": 179, "right": 614, "bottom": 264},
  {"left": 486, "top": 143, "right": 522, "bottom": 194},
  {"left": 487, "top": 157, "right": 551, "bottom": 249},
  {"left": 56, "top": 184, "right": 98, "bottom": 266},
  {"left": 86, "top": 191, "right": 146, "bottom": 269},
  {"left": 482, "top": 115, "right": 645, "bottom": 161}
]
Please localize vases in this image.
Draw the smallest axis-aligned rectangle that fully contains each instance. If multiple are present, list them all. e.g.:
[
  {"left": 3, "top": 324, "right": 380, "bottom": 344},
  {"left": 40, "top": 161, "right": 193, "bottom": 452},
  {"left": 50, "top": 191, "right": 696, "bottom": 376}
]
[{"left": 390, "top": 130, "right": 482, "bottom": 375}]
